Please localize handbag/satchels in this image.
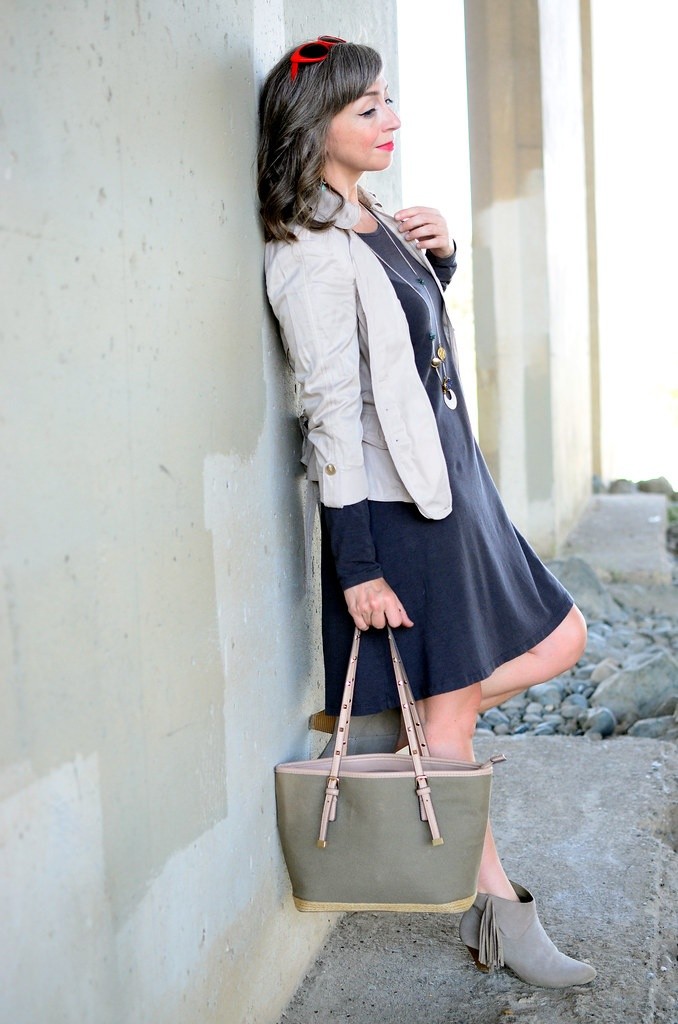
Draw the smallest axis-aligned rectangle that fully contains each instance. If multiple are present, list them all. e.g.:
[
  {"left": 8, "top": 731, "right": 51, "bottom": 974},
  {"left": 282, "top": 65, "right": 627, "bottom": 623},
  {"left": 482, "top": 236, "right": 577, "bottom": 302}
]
[{"left": 275, "top": 626, "right": 507, "bottom": 914}]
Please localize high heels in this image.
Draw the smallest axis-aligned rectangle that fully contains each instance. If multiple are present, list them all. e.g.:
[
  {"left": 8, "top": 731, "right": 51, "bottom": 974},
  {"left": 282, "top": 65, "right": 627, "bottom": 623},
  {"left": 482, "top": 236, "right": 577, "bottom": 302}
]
[
  {"left": 460, "top": 879, "right": 597, "bottom": 988},
  {"left": 309, "top": 708, "right": 402, "bottom": 759}
]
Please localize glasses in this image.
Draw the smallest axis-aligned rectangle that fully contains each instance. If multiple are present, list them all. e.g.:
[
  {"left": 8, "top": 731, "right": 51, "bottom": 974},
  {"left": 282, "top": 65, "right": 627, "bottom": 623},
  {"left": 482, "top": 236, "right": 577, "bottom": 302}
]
[{"left": 290, "top": 35, "right": 348, "bottom": 83}]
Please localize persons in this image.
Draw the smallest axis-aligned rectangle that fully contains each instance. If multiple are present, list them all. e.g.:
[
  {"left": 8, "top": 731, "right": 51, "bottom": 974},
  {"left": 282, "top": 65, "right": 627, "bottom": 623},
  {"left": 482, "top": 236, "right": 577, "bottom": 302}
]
[{"left": 258, "top": 33, "right": 598, "bottom": 989}]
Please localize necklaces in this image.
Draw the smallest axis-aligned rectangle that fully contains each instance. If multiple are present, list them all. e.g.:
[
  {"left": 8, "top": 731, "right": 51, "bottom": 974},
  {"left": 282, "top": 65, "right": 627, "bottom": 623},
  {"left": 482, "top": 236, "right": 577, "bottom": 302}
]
[{"left": 358, "top": 201, "right": 457, "bottom": 410}]
